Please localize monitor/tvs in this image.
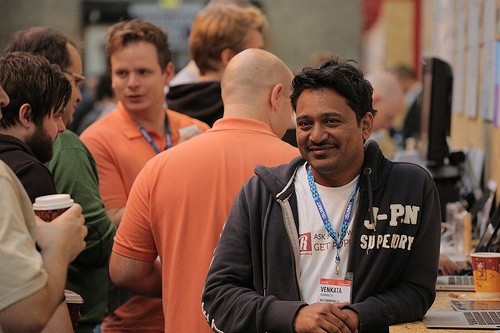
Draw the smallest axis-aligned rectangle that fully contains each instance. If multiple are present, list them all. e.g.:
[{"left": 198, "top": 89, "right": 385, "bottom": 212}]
[{"left": 418, "top": 57, "right": 452, "bottom": 168}]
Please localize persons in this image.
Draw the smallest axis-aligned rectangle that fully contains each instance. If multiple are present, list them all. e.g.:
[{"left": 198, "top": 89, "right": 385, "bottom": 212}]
[
  {"left": 0, "top": 85, "right": 88, "bottom": 333},
  {"left": 0, "top": 26, "right": 126, "bottom": 333},
  {"left": 163, "top": 0, "right": 269, "bottom": 127},
  {"left": 75, "top": 69, "right": 116, "bottom": 136},
  {"left": 79, "top": 19, "right": 211, "bottom": 333},
  {"left": 365, "top": 63, "right": 427, "bottom": 149},
  {"left": 0, "top": 51, "right": 74, "bottom": 333},
  {"left": 108, "top": 47, "right": 302, "bottom": 333},
  {"left": 199, "top": 57, "right": 441, "bottom": 333}
]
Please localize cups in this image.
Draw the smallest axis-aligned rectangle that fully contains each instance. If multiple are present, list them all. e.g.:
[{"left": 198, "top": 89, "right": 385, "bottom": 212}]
[
  {"left": 32, "top": 193, "right": 74, "bottom": 223},
  {"left": 470, "top": 252, "right": 500, "bottom": 298}
]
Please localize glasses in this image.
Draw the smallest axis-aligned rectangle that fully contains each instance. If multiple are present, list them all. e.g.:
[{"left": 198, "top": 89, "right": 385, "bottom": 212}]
[{"left": 60, "top": 68, "right": 86, "bottom": 89}]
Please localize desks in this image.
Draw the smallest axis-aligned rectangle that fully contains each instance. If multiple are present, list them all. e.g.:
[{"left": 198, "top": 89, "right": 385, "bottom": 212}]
[{"left": 389, "top": 276, "right": 500, "bottom": 333}]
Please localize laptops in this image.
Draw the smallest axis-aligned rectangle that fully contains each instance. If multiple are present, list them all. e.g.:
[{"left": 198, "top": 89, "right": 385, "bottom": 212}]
[{"left": 424, "top": 203, "right": 500, "bottom": 332}]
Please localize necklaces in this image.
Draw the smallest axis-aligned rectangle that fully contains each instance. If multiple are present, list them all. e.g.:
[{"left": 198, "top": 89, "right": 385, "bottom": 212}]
[{"left": 131, "top": 116, "right": 172, "bottom": 153}]
